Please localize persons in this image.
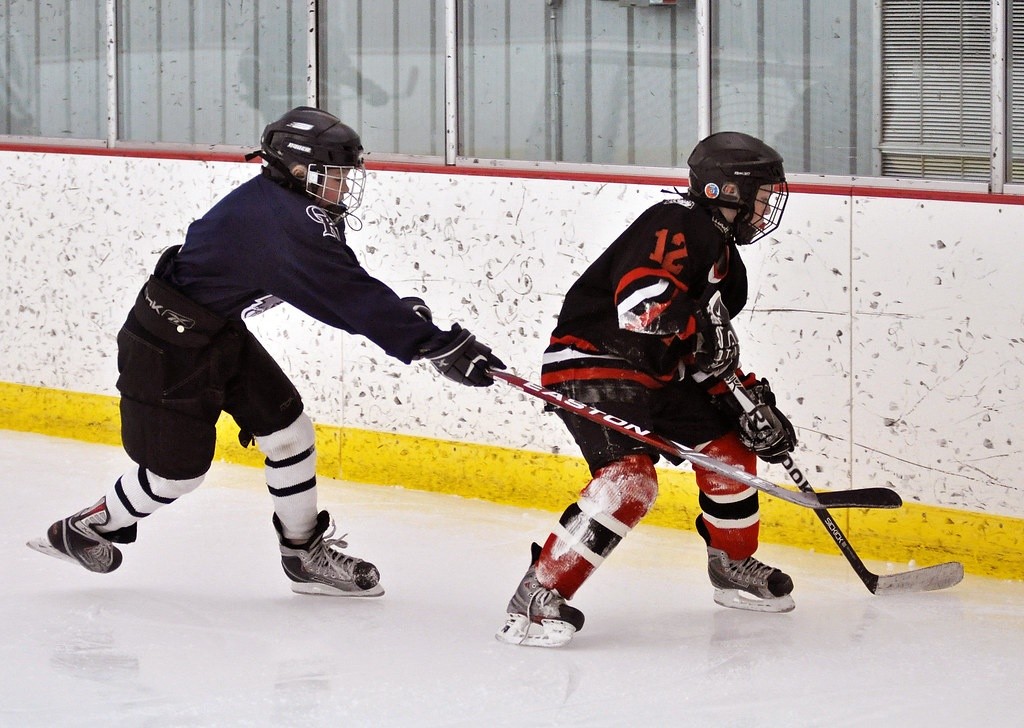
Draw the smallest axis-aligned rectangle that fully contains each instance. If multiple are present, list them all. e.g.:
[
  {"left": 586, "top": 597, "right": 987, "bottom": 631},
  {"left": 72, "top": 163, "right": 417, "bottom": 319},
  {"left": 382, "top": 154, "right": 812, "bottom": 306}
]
[
  {"left": 239, "top": 0, "right": 389, "bottom": 124},
  {"left": 26, "top": 106, "right": 507, "bottom": 597},
  {"left": 495, "top": 132, "right": 798, "bottom": 647}
]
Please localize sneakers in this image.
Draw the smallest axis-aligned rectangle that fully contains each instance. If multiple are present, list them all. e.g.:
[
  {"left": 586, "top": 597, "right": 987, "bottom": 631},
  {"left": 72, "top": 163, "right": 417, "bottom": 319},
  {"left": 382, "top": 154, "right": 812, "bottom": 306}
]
[
  {"left": 495, "top": 542, "right": 585, "bottom": 646},
  {"left": 272, "top": 511, "right": 385, "bottom": 597},
  {"left": 26, "top": 495, "right": 138, "bottom": 573},
  {"left": 695, "top": 513, "right": 796, "bottom": 612}
]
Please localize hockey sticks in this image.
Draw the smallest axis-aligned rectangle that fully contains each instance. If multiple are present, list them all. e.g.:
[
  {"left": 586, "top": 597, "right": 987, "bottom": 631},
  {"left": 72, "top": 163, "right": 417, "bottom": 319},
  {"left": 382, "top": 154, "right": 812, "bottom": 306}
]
[
  {"left": 483, "top": 364, "right": 903, "bottom": 509},
  {"left": 724, "top": 372, "right": 964, "bottom": 595}
]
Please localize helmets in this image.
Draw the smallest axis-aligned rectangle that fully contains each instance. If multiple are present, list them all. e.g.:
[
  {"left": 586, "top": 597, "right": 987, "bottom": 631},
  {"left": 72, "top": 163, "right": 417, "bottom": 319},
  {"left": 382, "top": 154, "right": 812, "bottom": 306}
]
[
  {"left": 687, "top": 131, "right": 788, "bottom": 245},
  {"left": 260, "top": 105, "right": 366, "bottom": 225}
]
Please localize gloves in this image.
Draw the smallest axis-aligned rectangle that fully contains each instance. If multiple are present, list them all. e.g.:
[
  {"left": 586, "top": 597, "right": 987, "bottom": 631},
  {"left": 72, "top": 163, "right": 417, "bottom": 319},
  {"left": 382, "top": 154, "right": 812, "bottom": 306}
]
[
  {"left": 687, "top": 283, "right": 739, "bottom": 379},
  {"left": 418, "top": 322, "right": 507, "bottom": 387},
  {"left": 400, "top": 296, "right": 432, "bottom": 324},
  {"left": 725, "top": 378, "right": 797, "bottom": 464}
]
